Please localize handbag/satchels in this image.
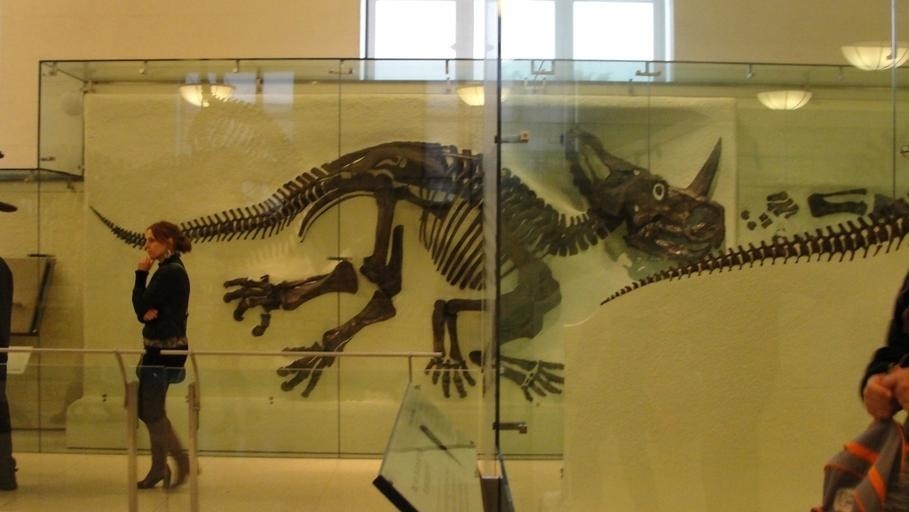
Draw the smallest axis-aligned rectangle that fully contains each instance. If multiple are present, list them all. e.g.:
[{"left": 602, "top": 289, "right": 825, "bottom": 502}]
[
  {"left": 136, "top": 353, "right": 186, "bottom": 383},
  {"left": 811, "top": 410, "right": 909, "bottom": 512}
]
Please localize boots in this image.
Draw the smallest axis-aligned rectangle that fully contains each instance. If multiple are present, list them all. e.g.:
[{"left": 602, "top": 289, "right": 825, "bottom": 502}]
[{"left": 137, "top": 448, "right": 189, "bottom": 489}]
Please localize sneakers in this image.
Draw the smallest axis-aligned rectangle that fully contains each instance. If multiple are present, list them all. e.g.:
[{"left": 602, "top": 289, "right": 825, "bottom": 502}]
[{"left": 0, "top": 458, "right": 17, "bottom": 491}]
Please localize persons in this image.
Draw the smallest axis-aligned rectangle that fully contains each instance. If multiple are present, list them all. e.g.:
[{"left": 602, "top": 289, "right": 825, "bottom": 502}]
[
  {"left": 808, "top": 269, "right": 909, "bottom": 512},
  {"left": 0, "top": 200, "right": 21, "bottom": 492},
  {"left": 130, "top": 219, "right": 199, "bottom": 491}
]
